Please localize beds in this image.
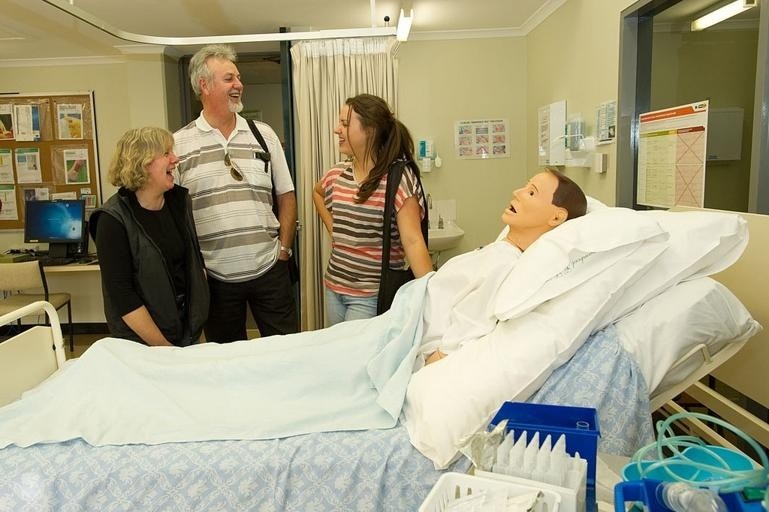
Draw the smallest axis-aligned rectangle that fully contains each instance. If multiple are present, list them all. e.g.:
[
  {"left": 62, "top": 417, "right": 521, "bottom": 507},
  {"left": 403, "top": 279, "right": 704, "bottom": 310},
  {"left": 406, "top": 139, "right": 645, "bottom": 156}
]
[{"left": 0, "top": 193, "right": 763, "bottom": 512}]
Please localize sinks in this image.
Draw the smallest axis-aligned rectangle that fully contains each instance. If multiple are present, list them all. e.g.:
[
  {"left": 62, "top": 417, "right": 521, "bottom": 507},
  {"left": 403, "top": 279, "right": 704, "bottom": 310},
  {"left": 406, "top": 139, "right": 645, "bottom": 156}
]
[{"left": 428, "top": 222, "right": 464, "bottom": 251}]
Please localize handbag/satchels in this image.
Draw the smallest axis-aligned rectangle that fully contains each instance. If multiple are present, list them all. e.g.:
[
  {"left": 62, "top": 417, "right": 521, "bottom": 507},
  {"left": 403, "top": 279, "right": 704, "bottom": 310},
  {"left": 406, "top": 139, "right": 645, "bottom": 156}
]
[{"left": 377, "top": 266, "right": 415, "bottom": 316}]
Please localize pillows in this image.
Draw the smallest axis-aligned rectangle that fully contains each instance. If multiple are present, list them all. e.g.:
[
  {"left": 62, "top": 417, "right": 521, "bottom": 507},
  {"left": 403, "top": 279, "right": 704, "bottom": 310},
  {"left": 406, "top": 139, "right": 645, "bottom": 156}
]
[
  {"left": 398, "top": 231, "right": 668, "bottom": 465},
  {"left": 490, "top": 204, "right": 663, "bottom": 324}
]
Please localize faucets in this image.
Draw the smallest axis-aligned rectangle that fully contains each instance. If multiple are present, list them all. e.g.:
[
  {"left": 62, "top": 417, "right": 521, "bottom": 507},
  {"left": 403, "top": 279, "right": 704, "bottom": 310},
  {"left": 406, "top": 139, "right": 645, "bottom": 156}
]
[{"left": 425, "top": 192, "right": 432, "bottom": 211}]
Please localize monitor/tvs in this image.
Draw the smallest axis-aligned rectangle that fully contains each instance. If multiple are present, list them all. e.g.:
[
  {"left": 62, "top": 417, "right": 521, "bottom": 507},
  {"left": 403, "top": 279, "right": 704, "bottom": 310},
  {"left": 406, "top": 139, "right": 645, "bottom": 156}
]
[{"left": 24, "top": 200, "right": 85, "bottom": 265}]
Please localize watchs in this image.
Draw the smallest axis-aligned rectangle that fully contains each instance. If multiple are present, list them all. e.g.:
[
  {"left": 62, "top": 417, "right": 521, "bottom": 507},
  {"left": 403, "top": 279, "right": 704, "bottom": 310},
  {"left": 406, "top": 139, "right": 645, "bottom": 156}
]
[{"left": 280, "top": 245, "right": 293, "bottom": 257}]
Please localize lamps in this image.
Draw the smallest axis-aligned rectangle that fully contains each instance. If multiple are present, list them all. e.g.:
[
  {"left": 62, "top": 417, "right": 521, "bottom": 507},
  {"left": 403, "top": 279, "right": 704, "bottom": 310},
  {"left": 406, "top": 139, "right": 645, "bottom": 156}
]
[
  {"left": 397, "top": 9, "right": 414, "bottom": 42},
  {"left": 691, "top": 0, "right": 757, "bottom": 32}
]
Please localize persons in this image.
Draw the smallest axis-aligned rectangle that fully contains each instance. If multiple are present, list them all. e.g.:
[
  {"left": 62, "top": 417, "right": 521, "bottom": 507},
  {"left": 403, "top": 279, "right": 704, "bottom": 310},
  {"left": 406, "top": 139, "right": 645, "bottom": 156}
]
[
  {"left": 163, "top": 42, "right": 299, "bottom": 344},
  {"left": 311, "top": 92, "right": 434, "bottom": 326},
  {"left": 419, "top": 166, "right": 588, "bottom": 368},
  {"left": 89, "top": 126, "right": 212, "bottom": 347}
]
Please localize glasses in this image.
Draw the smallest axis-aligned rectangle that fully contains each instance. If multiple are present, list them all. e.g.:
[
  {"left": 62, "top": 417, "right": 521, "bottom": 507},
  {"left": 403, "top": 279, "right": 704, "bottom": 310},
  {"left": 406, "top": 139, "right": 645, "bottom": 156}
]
[{"left": 223, "top": 152, "right": 244, "bottom": 181}]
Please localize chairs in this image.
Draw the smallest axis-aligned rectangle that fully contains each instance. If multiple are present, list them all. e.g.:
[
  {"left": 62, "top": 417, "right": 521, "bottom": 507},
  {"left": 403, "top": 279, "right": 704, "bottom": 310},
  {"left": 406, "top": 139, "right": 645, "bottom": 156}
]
[{"left": 0, "top": 260, "right": 74, "bottom": 354}]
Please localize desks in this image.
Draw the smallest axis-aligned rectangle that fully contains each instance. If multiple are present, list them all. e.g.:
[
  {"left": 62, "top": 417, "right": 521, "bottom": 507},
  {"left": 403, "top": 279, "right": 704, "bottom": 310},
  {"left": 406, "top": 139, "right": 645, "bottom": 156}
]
[{"left": 0, "top": 252, "right": 106, "bottom": 336}]
